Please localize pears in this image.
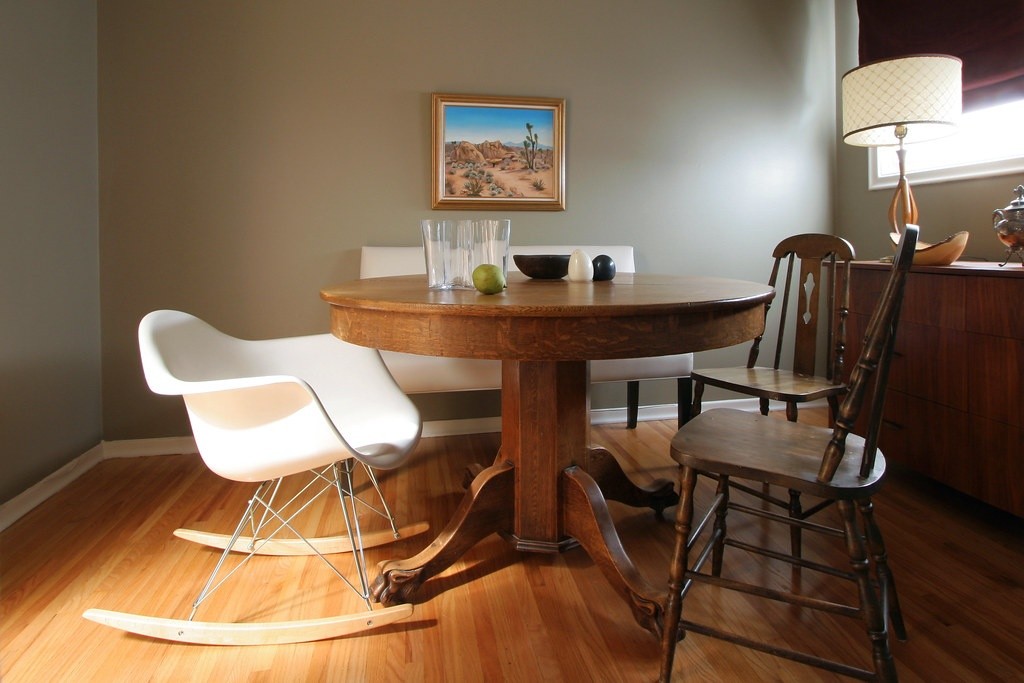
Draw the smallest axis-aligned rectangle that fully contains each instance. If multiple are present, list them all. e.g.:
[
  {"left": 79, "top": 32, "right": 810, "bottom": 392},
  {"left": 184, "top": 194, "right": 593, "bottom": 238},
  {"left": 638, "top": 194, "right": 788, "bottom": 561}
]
[{"left": 472, "top": 264, "right": 508, "bottom": 295}]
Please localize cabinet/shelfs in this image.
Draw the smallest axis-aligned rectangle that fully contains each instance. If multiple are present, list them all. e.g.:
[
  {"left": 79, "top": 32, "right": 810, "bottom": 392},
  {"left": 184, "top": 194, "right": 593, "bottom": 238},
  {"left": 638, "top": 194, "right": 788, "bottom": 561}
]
[{"left": 822, "top": 259, "right": 1024, "bottom": 518}]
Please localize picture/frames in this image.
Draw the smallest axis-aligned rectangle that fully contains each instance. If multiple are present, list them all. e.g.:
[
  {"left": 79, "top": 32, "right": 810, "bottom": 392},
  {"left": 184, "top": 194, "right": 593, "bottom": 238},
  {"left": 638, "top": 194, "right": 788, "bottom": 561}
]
[{"left": 432, "top": 93, "right": 566, "bottom": 211}]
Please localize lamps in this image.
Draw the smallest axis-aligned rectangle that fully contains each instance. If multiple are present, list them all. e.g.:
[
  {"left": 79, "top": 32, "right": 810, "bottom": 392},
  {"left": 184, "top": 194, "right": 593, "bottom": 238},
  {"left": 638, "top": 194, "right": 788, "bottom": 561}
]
[{"left": 842, "top": 54, "right": 962, "bottom": 262}]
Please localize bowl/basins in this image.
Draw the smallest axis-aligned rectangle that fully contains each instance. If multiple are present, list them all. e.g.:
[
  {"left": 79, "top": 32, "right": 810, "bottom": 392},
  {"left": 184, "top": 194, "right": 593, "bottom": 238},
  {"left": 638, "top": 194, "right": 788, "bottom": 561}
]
[{"left": 513, "top": 255, "right": 571, "bottom": 280}]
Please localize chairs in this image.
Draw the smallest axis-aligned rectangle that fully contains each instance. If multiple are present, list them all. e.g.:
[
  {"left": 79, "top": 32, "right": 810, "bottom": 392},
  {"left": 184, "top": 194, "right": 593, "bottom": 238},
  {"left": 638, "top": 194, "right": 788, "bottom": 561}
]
[
  {"left": 83, "top": 310, "right": 423, "bottom": 646},
  {"left": 660, "top": 223, "right": 915, "bottom": 683}
]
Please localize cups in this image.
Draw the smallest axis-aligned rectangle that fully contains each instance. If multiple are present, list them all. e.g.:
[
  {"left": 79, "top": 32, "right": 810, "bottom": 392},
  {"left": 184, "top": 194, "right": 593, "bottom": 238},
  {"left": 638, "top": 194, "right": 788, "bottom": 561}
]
[
  {"left": 441, "top": 218, "right": 475, "bottom": 289},
  {"left": 421, "top": 219, "right": 453, "bottom": 290},
  {"left": 473, "top": 219, "right": 511, "bottom": 286}
]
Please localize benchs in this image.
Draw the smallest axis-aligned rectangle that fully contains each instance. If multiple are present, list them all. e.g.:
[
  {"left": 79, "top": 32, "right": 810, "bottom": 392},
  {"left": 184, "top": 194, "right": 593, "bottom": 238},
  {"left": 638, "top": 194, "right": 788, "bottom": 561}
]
[{"left": 361, "top": 246, "right": 693, "bottom": 429}]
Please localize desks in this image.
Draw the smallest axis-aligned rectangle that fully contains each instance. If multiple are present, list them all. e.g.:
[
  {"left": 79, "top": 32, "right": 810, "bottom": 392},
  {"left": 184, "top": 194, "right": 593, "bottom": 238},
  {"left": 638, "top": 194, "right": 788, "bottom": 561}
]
[{"left": 321, "top": 274, "right": 777, "bottom": 645}]
